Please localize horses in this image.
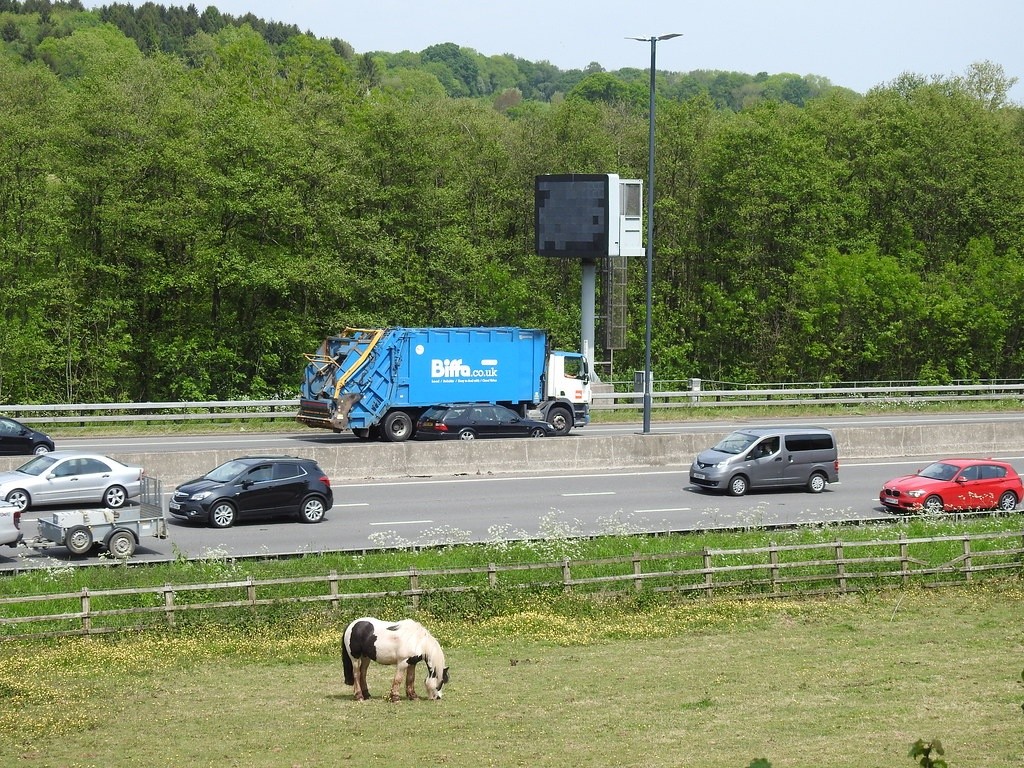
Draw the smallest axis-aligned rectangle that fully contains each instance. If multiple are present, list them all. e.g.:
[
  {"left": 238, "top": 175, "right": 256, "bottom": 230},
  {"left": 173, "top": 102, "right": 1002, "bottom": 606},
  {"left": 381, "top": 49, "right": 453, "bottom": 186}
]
[{"left": 341, "top": 617, "right": 449, "bottom": 704}]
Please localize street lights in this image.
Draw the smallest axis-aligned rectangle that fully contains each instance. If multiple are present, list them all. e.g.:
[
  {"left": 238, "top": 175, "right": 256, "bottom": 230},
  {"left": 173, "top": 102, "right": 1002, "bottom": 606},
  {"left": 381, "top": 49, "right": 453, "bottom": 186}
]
[{"left": 624, "top": 33, "right": 687, "bottom": 433}]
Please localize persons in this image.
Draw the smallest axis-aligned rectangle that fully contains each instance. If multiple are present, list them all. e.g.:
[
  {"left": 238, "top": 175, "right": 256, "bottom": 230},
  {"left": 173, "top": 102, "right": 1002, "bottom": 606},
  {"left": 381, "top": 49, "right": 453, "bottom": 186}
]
[
  {"left": 263, "top": 467, "right": 272, "bottom": 480},
  {"left": 766, "top": 444, "right": 773, "bottom": 454}
]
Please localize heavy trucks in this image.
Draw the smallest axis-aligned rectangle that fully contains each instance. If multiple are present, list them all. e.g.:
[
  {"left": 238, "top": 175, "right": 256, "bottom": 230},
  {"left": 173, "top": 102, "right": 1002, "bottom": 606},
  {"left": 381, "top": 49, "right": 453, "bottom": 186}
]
[{"left": 295, "top": 325, "right": 593, "bottom": 442}]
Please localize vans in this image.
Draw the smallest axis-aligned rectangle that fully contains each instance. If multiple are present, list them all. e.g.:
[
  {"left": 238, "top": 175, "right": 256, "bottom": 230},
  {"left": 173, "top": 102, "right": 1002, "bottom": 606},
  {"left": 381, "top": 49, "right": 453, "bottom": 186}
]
[{"left": 689, "top": 425, "right": 839, "bottom": 497}]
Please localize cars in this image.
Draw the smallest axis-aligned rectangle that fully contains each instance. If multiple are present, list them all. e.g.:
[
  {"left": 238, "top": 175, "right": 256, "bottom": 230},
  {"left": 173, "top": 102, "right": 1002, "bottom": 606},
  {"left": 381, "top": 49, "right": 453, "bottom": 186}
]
[
  {"left": 879, "top": 455, "right": 1024, "bottom": 521},
  {"left": 169, "top": 454, "right": 334, "bottom": 528},
  {"left": 0, "top": 449, "right": 147, "bottom": 514},
  {"left": 0, "top": 415, "right": 55, "bottom": 456}
]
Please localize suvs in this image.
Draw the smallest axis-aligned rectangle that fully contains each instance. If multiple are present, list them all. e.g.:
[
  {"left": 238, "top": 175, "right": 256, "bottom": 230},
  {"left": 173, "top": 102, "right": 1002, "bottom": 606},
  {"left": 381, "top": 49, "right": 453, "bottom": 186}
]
[{"left": 413, "top": 402, "right": 557, "bottom": 441}]
